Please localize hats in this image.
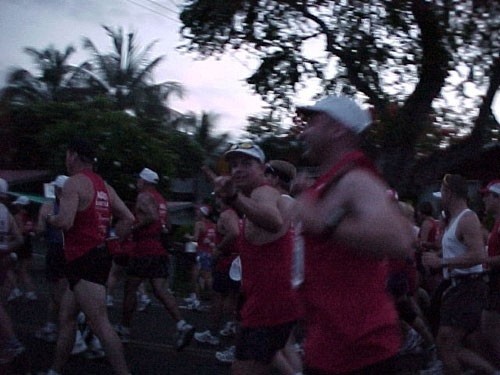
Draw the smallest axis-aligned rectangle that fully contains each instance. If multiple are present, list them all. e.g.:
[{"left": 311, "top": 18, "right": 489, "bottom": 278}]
[
  {"left": 294, "top": 95, "right": 371, "bottom": 136},
  {"left": 223, "top": 142, "right": 266, "bottom": 165},
  {"left": 68, "top": 134, "right": 98, "bottom": 160},
  {"left": 50, "top": 174, "right": 70, "bottom": 189},
  {"left": 479, "top": 178, "right": 500, "bottom": 194},
  {"left": 139, "top": 167, "right": 159, "bottom": 184}
]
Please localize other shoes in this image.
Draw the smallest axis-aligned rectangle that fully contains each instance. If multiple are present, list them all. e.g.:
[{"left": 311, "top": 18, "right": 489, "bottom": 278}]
[
  {"left": 216, "top": 346, "right": 236, "bottom": 363},
  {"left": 7, "top": 287, "right": 22, "bottom": 302},
  {"left": 193, "top": 329, "right": 220, "bottom": 345},
  {"left": 72, "top": 331, "right": 88, "bottom": 355},
  {"left": 0, "top": 340, "right": 25, "bottom": 365},
  {"left": 136, "top": 293, "right": 152, "bottom": 312},
  {"left": 416, "top": 345, "right": 444, "bottom": 375},
  {"left": 219, "top": 320, "right": 235, "bottom": 336},
  {"left": 399, "top": 331, "right": 423, "bottom": 354},
  {"left": 175, "top": 320, "right": 194, "bottom": 350},
  {"left": 114, "top": 325, "right": 131, "bottom": 344},
  {"left": 79, "top": 335, "right": 105, "bottom": 359},
  {"left": 106, "top": 294, "right": 115, "bottom": 308},
  {"left": 24, "top": 290, "right": 38, "bottom": 301},
  {"left": 34, "top": 322, "right": 57, "bottom": 343}
]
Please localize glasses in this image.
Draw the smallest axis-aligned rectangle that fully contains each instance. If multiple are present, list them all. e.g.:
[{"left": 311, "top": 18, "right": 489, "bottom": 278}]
[{"left": 229, "top": 142, "right": 259, "bottom": 152}]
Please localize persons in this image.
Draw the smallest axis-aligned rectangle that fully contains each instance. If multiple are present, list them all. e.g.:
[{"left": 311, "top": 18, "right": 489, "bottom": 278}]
[{"left": 0, "top": 96, "right": 500, "bottom": 375}]
